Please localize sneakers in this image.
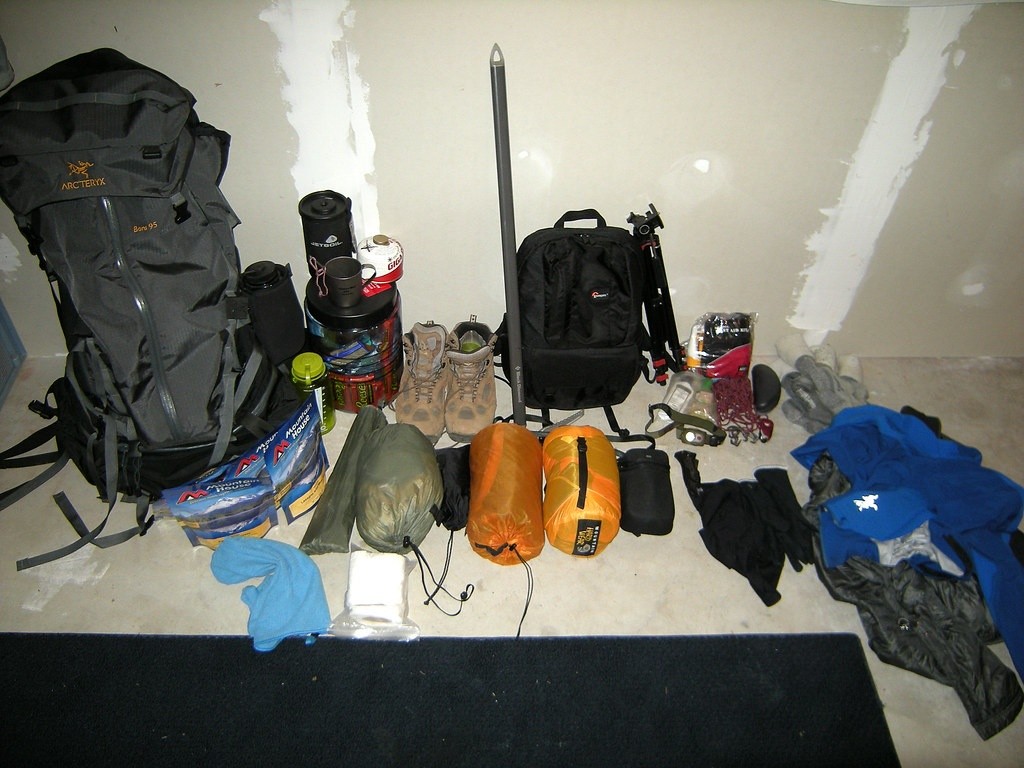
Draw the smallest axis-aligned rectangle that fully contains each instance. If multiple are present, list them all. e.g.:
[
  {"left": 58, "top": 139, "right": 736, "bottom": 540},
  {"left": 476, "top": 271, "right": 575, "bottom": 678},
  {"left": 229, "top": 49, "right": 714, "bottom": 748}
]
[
  {"left": 388, "top": 320, "right": 449, "bottom": 443},
  {"left": 444, "top": 314, "right": 498, "bottom": 442}
]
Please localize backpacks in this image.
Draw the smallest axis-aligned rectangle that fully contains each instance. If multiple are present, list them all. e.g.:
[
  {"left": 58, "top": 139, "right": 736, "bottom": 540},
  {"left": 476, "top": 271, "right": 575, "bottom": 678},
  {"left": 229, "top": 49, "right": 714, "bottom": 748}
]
[
  {"left": 494, "top": 208, "right": 652, "bottom": 410},
  {"left": 0, "top": 48, "right": 307, "bottom": 572}
]
